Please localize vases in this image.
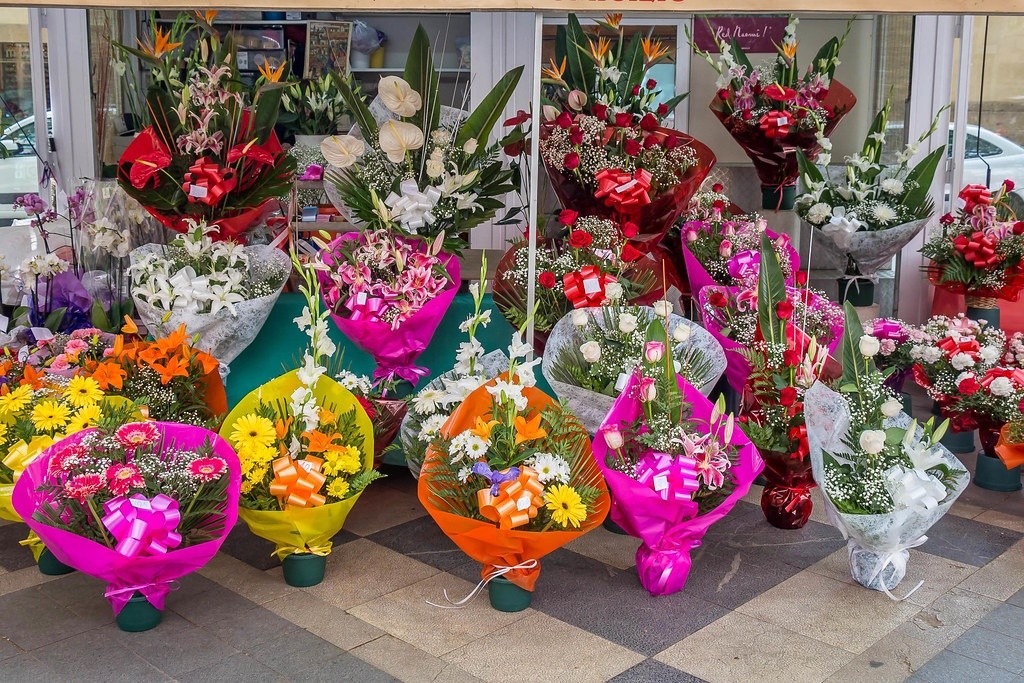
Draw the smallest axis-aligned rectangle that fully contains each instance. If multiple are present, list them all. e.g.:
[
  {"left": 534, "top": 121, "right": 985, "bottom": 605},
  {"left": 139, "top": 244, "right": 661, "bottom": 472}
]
[
  {"left": 110, "top": 586, "right": 164, "bottom": 633},
  {"left": 488, "top": 575, "right": 533, "bottom": 613}
]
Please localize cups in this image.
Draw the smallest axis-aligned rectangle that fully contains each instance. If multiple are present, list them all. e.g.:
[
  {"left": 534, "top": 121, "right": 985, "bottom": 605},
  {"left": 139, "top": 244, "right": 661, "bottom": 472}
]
[
  {"left": 351, "top": 48, "right": 370, "bottom": 69},
  {"left": 370, "top": 47, "right": 384, "bottom": 68}
]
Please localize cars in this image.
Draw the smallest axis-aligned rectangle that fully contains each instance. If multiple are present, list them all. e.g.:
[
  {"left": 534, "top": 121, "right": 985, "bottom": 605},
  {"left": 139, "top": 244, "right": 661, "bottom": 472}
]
[
  {"left": 1, "top": 106, "right": 118, "bottom": 227},
  {"left": 882, "top": 118, "right": 1023, "bottom": 202}
]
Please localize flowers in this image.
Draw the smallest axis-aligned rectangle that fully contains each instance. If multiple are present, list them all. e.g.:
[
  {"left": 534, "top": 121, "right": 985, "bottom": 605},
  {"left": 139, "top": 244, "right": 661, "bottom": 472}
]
[{"left": 1, "top": 11, "right": 1023, "bottom": 594}]
